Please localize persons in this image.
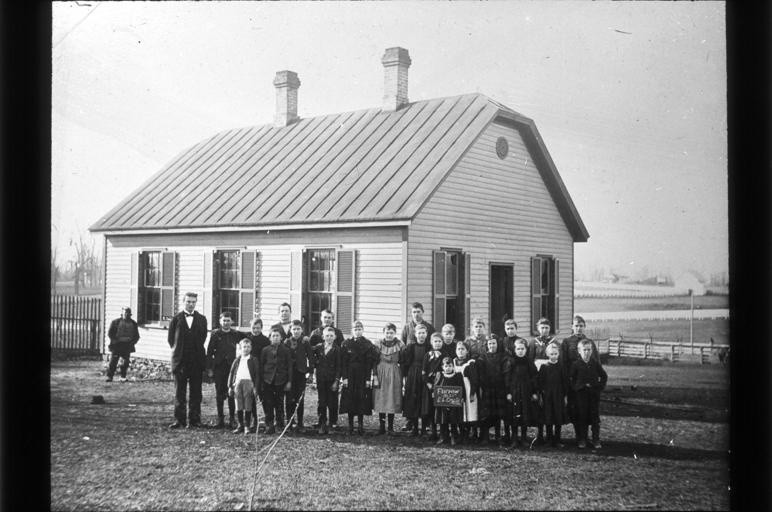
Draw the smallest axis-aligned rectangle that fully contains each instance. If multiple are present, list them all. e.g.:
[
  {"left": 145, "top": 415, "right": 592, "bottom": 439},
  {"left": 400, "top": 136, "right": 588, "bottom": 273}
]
[
  {"left": 206, "top": 311, "right": 246, "bottom": 430},
  {"left": 371, "top": 322, "right": 406, "bottom": 438},
  {"left": 259, "top": 327, "right": 293, "bottom": 435},
  {"left": 226, "top": 336, "right": 260, "bottom": 436},
  {"left": 167, "top": 292, "right": 208, "bottom": 431},
  {"left": 285, "top": 319, "right": 314, "bottom": 435},
  {"left": 309, "top": 310, "right": 341, "bottom": 430},
  {"left": 399, "top": 300, "right": 607, "bottom": 450},
  {"left": 315, "top": 326, "right": 342, "bottom": 435},
  {"left": 242, "top": 318, "right": 269, "bottom": 415},
  {"left": 267, "top": 302, "right": 294, "bottom": 342},
  {"left": 104, "top": 305, "right": 140, "bottom": 383},
  {"left": 341, "top": 320, "right": 373, "bottom": 436}
]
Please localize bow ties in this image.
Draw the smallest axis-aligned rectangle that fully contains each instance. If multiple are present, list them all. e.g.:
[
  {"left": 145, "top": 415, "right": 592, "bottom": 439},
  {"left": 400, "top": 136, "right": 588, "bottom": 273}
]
[{"left": 184, "top": 312, "right": 195, "bottom": 318}]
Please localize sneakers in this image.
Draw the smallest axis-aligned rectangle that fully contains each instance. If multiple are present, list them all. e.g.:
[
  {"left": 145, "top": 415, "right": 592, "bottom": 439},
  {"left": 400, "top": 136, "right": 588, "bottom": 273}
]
[
  {"left": 228, "top": 417, "right": 238, "bottom": 427},
  {"left": 317, "top": 422, "right": 327, "bottom": 435},
  {"left": 212, "top": 419, "right": 226, "bottom": 429},
  {"left": 168, "top": 419, "right": 188, "bottom": 429},
  {"left": 578, "top": 440, "right": 588, "bottom": 449},
  {"left": 244, "top": 426, "right": 251, "bottom": 435},
  {"left": 347, "top": 421, "right": 566, "bottom": 450},
  {"left": 249, "top": 417, "right": 307, "bottom": 435},
  {"left": 327, "top": 423, "right": 334, "bottom": 435},
  {"left": 232, "top": 423, "right": 244, "bottom": 434},
  {"left": 591, "top": 441, "right": 602, "bottom": 449},
  {"left": 119, "top": 376, "right": 129, "bottom": 381},
  {"left": 188, "top": 418, "right": 208, "bottom": 429},
  {"left": 105, "top": 376, "right": 113, "bottom": 382}
]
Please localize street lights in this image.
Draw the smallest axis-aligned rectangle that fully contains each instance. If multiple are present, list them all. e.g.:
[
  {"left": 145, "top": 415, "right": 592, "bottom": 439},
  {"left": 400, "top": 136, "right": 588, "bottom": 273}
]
[{"left": 687, "top": 288, "right": 694, "bottom": 355}]
